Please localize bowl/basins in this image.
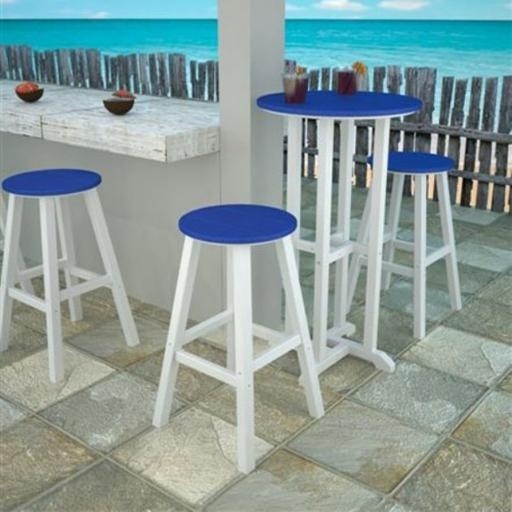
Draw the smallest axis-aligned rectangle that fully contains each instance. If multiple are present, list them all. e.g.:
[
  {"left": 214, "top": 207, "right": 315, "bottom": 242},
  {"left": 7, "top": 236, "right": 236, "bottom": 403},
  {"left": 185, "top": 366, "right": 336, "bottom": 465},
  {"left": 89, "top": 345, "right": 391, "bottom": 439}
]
[
  {"left": 16, "top": 88, "right": 45, "bottom": 102},
  {"left": 102, "top": 98, "right": 134, "bottom": 115}
]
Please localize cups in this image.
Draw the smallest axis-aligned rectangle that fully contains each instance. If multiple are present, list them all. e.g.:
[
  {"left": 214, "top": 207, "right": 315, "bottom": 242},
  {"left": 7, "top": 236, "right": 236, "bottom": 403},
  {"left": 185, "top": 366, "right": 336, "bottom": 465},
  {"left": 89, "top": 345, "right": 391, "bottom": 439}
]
[
  {"left": 282, "top": 73, "right": 308, "bottom": 103},
  {"left": 338, "top": 71, "right": 356, "bottom": 96}
]
